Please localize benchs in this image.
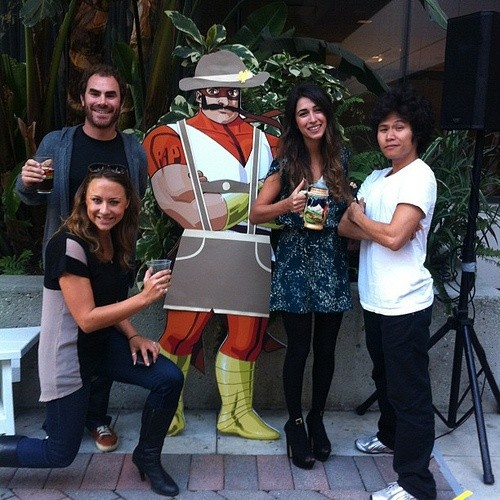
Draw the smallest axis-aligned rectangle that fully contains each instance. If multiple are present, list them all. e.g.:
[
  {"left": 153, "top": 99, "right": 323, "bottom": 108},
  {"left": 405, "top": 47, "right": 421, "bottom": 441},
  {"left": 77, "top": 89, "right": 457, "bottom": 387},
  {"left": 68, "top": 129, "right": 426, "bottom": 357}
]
[{"left": 0, "top": 324, "right": 41, "bottom": 436}]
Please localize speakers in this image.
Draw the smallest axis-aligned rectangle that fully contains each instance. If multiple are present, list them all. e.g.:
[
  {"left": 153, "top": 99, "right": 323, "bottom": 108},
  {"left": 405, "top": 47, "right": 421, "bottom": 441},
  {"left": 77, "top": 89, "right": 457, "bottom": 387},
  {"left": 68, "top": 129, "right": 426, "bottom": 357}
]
[{"left": 440, "top": 10, "right": 500, "bottom": 131}]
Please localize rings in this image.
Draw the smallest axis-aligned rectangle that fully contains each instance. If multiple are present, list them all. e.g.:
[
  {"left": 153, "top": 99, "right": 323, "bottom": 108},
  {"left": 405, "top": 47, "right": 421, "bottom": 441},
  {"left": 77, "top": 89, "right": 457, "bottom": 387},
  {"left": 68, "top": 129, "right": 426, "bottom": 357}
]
[
  {"left": 165, "top": 288, "right": 167, "bottom": 292},
  {"left": 132, "top": 353, "right": 135, "bottom": 356},
  {"left": 153, "top": 342, "right": 155, "bottom": 344}
]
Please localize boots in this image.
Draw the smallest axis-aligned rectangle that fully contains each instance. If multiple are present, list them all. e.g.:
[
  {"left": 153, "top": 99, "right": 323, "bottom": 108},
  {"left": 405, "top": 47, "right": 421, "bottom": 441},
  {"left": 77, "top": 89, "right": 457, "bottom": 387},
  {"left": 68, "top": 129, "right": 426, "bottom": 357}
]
[
  {"left": 0, "top": 435, "right": 25, "bottom": 468},
  {"left": 132, "top": 397, "right": 180, "bottom": 497}
]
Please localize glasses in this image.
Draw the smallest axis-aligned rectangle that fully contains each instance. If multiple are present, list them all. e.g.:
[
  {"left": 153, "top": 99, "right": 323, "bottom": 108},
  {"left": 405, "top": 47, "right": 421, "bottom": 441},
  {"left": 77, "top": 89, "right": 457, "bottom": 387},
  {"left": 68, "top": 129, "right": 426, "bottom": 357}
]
[{"left": 87, "top": 163, "right": 129, "bottom": 182}]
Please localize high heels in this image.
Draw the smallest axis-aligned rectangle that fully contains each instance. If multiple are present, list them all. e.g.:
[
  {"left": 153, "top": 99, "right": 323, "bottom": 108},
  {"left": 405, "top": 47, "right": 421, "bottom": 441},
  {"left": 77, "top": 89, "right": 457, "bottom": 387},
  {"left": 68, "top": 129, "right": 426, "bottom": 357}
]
[
  {"left": 305, "top": 411, "right": 331, "bottom": 461},
  {"left": 284, "top": 417, "right": 315, "bottom": 470}
]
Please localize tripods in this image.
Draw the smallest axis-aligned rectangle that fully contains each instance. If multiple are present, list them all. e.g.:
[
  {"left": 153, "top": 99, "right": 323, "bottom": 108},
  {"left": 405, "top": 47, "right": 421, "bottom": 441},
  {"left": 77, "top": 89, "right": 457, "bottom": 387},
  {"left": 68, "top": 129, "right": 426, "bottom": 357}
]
[{"left": 355, "top": 129, "right": 500, "bottom": 484}]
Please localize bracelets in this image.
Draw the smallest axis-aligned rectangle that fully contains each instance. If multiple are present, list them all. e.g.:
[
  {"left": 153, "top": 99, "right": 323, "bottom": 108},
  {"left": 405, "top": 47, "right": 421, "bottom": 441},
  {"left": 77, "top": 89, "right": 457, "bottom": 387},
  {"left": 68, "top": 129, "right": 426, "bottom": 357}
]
[{"left": 129, "top": 335, "right": 138, "bottom": 341}]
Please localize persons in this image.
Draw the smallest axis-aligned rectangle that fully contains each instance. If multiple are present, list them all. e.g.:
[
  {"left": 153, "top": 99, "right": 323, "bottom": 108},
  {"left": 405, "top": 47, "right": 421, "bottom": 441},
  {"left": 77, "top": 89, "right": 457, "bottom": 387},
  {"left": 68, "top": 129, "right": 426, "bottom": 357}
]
[
  {"left": 0, "top": 163, "right": 184, "bottom": 497},
  {"left": 337, "top": 87, "right": 437, "bottom": 500},
  {"left": 15, "top": 64, "right": 148, "bottom": 452},
  {"left": 249, "top": 82, "right": 355, "bottom": 470}
]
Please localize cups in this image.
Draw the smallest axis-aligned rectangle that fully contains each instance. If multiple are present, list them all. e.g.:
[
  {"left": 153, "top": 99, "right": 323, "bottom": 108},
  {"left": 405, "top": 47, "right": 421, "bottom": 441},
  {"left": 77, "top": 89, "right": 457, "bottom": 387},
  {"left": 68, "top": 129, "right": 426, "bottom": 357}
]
[
  {"left": 33, "top": 157, "right": 55, "bottom": 194},
  {"left": 146, "top": 260, "right": 171, "bottom": 284},
  {"left": 299, "top": 179, "right": 329, "bottom": 230}
]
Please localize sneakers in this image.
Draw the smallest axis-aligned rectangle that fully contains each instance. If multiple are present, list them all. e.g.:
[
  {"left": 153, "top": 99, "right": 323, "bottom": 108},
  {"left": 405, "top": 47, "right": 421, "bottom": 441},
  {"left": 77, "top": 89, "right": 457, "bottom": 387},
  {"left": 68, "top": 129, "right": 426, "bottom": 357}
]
[
  {"left": 95, "top": 424, "right": 118, "bottom": 451},
  {"left": 370, "top": 481, "right": 416, "bottom": 500},
  {"left": 355, "top": 434, "right": 394, "bottom": 454}
]
